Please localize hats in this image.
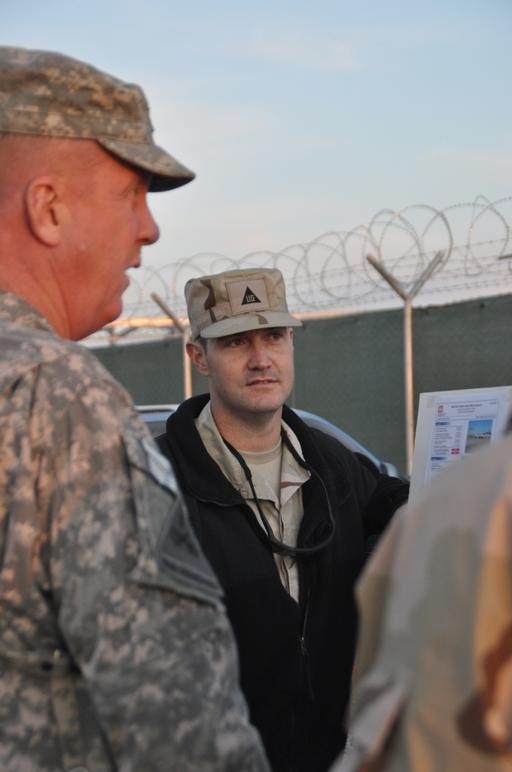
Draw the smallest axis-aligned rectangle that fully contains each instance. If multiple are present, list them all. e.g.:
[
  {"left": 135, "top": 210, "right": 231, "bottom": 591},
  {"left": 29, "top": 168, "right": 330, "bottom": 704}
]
[
  {"left": 0, "top": 47, "right": 199, "bottom": 191},
  {"left": 184, "top": 267, "right": 306, "bottom": 340}
]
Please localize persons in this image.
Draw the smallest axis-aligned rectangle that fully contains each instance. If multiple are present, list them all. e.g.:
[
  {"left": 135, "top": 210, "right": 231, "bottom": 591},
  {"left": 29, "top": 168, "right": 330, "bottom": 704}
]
[
  {"left": 1, "top": 45, "right": 274, "bottom": 772},
  {"left": 152, "top": 266, "right": 413, "bottom": 771}
]
